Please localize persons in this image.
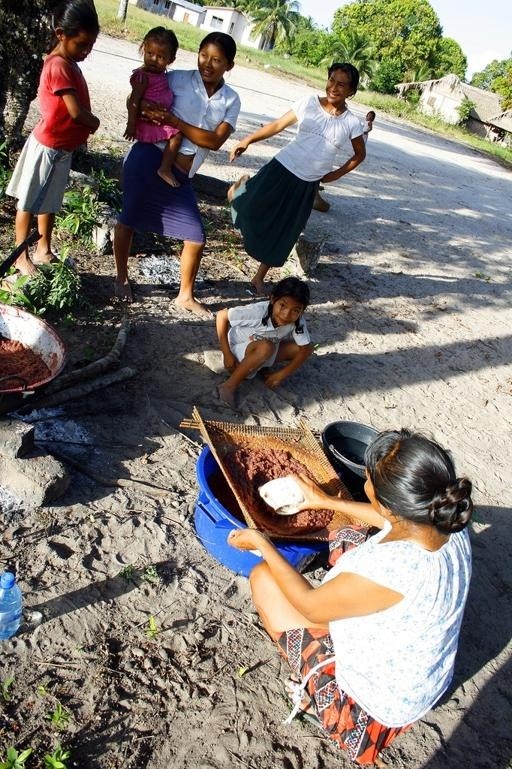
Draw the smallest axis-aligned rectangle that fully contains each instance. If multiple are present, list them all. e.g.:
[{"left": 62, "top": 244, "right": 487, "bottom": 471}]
[
  {"left": 362, "top": 110, "right": 375, "bottom": 144},
  {"left": 213, "top": 276, "right": 313, "bottom": 405},
  {"left": 123, "top": 25, "right": 184, "bottom": 188},
  {"left": 227, "top": 62, "right": 366, "bottom": 298},
  {"left": 227, "top": 427, "right": 474, "bottom": 766},
  {"left": 5, "top": 1, "right": 100, "bottom": 278},
  {"left": 114, "top": 32, "right": 240, "bottom": 317}
]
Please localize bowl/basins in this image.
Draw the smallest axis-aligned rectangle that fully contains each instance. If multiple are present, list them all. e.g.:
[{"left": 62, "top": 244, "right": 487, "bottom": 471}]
[
  {"left": 1, "top": 305, "right": 67, "bottom": 395},
  {"left": 256, "top": 476, "right": 305, "bottom": 517},
  {"left": 322, "top": 420, "right": 383, "bottom": 478}
]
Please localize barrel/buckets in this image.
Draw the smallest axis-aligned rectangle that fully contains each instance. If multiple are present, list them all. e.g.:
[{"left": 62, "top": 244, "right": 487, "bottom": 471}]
[{"left": 321, "top": 418, "right": 388, "bottom": 505}]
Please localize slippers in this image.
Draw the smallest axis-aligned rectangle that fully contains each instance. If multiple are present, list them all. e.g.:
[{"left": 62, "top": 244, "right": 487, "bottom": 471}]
[{"left": 283, "top": 676, "right": 323, "bottom": 731}]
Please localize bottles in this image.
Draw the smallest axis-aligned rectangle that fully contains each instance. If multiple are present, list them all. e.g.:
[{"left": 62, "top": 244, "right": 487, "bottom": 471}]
[{"left": 0, "top": 572, "right": 24, "bottom": 641}]
